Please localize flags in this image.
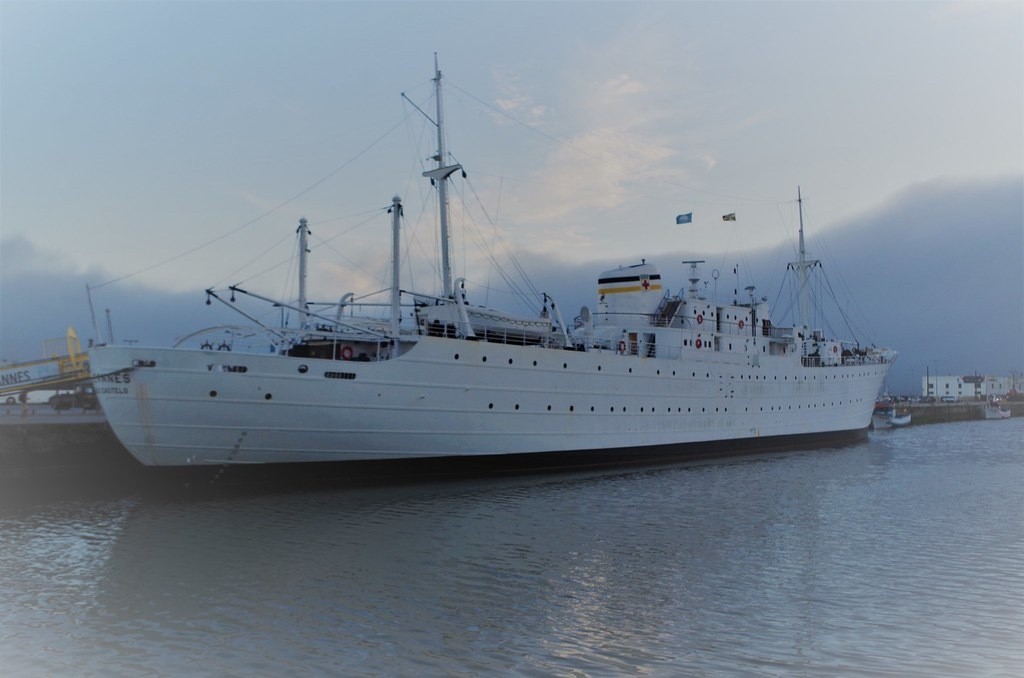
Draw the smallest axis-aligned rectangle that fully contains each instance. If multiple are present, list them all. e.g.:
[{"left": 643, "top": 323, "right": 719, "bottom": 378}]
[
  {"left": 676, "top": 213, "right": 692, "bottom": 224},
  {"left": 722, "top": 213, "right": 736, "bottom": 221}
]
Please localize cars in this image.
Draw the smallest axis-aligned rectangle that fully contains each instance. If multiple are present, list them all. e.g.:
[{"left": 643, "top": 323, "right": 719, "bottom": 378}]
[{"left": 874, "top": 395, "right": 957, "bottom": 404}]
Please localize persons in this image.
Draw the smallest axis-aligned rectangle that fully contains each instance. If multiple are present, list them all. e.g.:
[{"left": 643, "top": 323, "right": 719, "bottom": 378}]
[{"left": 20, "top": 392, "right": 30, "bottom": 415}]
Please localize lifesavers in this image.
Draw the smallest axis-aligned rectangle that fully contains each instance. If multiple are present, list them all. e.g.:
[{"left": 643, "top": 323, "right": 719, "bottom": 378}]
[
  {"left": 697, "top": 315, "right": 703, "bottom": 324},
  {"left": 739, "top": 320, "right": 744, "bottom": 329},
  {"left": 342, "top": 346, "right": 354, "bottom": 360},
  {"left": 833, "top": 346, "right": 837, "bottom": 352},
  {"left": 7, "top": 396, "right": 16, "bottom": 404},
  {"left": 619, "top": 341, "right": 626, "bottom": 353},
  {"left": 696, "top": 338, "right": 702, "bottom": 349}
]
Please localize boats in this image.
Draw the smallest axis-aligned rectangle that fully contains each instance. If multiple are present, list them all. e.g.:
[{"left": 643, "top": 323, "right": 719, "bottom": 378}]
[
  {"left": 871, "top": 403, "right": 911, "bottom": 430},
  {"left": 985, "top": 398, "right": 1011, "bottom": 420},
  {"left": 85, "top": 49, "right": 900, "bottom": 496}
]
